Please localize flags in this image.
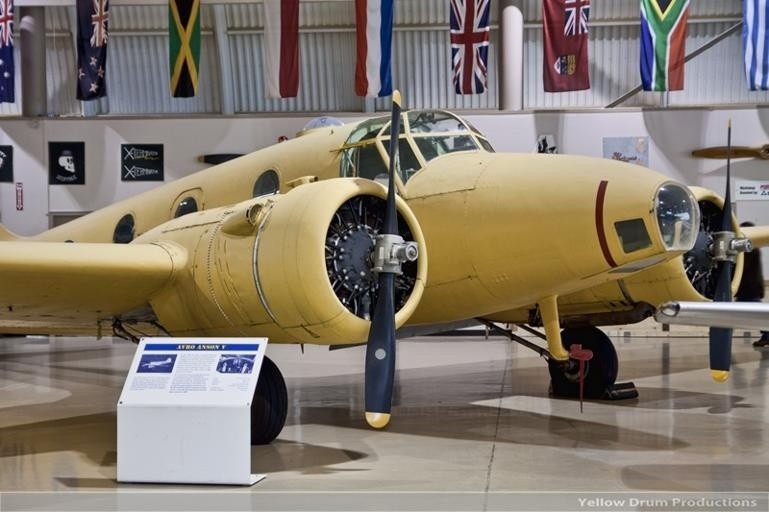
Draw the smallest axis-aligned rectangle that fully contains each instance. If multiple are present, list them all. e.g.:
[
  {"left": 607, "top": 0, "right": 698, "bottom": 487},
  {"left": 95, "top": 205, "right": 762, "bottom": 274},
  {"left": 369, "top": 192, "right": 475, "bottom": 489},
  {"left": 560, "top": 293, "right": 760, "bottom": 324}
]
[
  {"left": 639, "top": 0, "right": 692, "bottom": 91},
  {"left": 741, "top": 0, "right": 769, "bottom": 91},
  {"left": 0, "top": 0, "right": 15, "bottom": 103},
  {"left": 76, "top": 0, "right": 109, "bottom": 101},
  {"left": 168, "top": 0, "right": 201, "bottom": 98},
  {"left": 450, "top": 0, "right": 491, "bottom": 94},
  {"left": 263, "top": 0, "right": 299, "bottom": 99},
  {"left": 355, "top": 0, "right": 393, "bottom": 97},
  {"left": 541, "top": 0, "right": 590, "bottom": 92}
]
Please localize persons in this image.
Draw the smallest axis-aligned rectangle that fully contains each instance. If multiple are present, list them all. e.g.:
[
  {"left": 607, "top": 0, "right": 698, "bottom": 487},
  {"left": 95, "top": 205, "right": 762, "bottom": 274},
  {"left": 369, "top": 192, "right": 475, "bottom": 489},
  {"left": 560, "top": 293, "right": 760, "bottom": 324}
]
[{"left": 737, "top": 221, "right": 769, "bottom": 347}]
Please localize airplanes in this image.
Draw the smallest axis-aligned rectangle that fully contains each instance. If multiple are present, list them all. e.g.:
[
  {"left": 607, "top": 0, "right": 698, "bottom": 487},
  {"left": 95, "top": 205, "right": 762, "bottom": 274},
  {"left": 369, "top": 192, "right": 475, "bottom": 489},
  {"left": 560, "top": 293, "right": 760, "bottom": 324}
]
[{"left": 0, "top": 89, "right": 768, "bottom": 445}]
